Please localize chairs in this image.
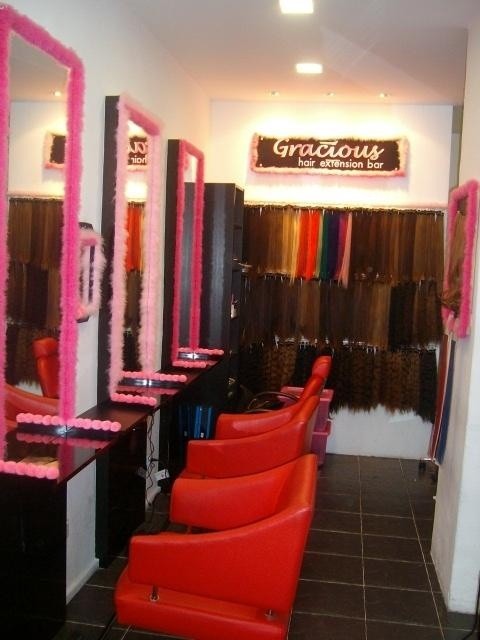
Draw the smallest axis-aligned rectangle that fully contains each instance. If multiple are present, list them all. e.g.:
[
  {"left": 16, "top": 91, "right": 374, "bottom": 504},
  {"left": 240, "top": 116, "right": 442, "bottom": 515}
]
[
  {"left": 5, "top": 378, "right": 59, "bottom": 432},
  {"left": 178, "top": 394, "right": 321, "bottom": 480},
  {"left": 33, "top": 338, "right": 60, "bottom": 398},
  {"left": 114, "top": 454, "right": 318, "bottom": 639},
  {"left": 216, "top": 355, "right": 332, "bottom": 439},
  {"left": 240, "top": 387, "right": 335, "bottom": 465}
]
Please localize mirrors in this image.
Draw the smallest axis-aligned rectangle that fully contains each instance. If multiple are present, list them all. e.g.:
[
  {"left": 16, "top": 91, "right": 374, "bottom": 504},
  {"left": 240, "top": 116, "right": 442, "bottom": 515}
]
[
  {"left": 97, "top": 94, "right": 165, "bottom": 405},
  {"left": 162, "top": 139, "right": 205, "bottom": 368},
  {"left": 1, "top": 3, "right": 85, "bottom": 477}
]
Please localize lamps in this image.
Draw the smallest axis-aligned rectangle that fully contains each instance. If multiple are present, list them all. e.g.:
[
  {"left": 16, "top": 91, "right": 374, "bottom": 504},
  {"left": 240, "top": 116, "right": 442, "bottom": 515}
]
[
  {"left": 278, "top": 0, "right": 314, "bottom": 15},
  {"left": 297, "top": 63, "right": 322, "bottom": 75}
]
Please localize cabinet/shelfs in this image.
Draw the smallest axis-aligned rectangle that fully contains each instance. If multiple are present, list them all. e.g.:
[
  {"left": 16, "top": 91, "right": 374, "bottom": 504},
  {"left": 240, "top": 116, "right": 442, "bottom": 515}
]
[
  {"left": 0, "top": 367, "right": 214, "bottom": 640},
  {"left": 205, "top": 182, "right": 245, "bottom": 402}
]
